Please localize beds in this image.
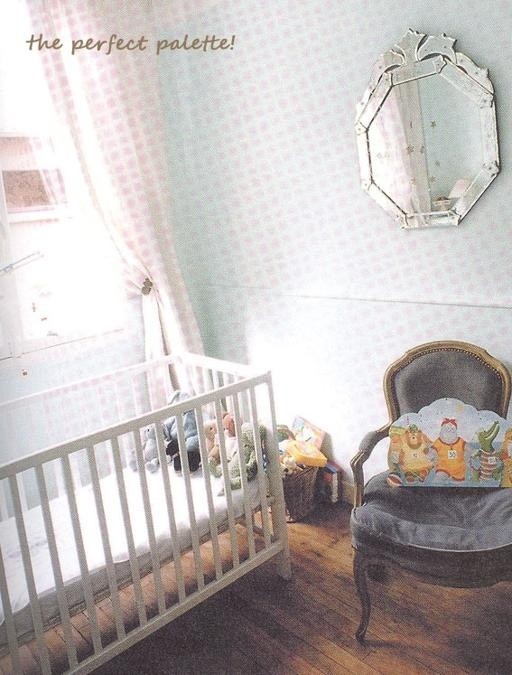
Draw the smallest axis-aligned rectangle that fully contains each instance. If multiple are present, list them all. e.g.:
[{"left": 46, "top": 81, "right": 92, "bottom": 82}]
[{"left": 0, "top": 351, "right": 292, "bottom": 674}]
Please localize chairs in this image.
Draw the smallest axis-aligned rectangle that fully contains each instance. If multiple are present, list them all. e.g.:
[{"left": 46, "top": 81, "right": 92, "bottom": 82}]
[{"left": 350, "top": 339, "right": 512, "bottom": 643}]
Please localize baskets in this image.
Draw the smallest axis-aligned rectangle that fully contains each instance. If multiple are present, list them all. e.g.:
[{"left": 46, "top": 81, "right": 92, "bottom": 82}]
[{"left": 284, "top": 467, "right": 319, "bottom": 523}]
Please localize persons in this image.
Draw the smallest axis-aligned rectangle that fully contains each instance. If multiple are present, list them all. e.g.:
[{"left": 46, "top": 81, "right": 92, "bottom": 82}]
[{"left": 128, "top": 423, "right": 169, "bottom": 472}]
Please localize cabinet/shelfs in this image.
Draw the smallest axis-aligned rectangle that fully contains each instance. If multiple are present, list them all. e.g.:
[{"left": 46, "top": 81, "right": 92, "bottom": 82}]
[{"left": 0, "top": 132, "right": 67, "bottom": 212}]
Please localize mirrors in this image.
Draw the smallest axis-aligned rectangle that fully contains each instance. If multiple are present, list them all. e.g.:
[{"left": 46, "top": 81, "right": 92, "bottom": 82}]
[{"left": 354, "top": 28, "right": 501, "bottom": 229}]
